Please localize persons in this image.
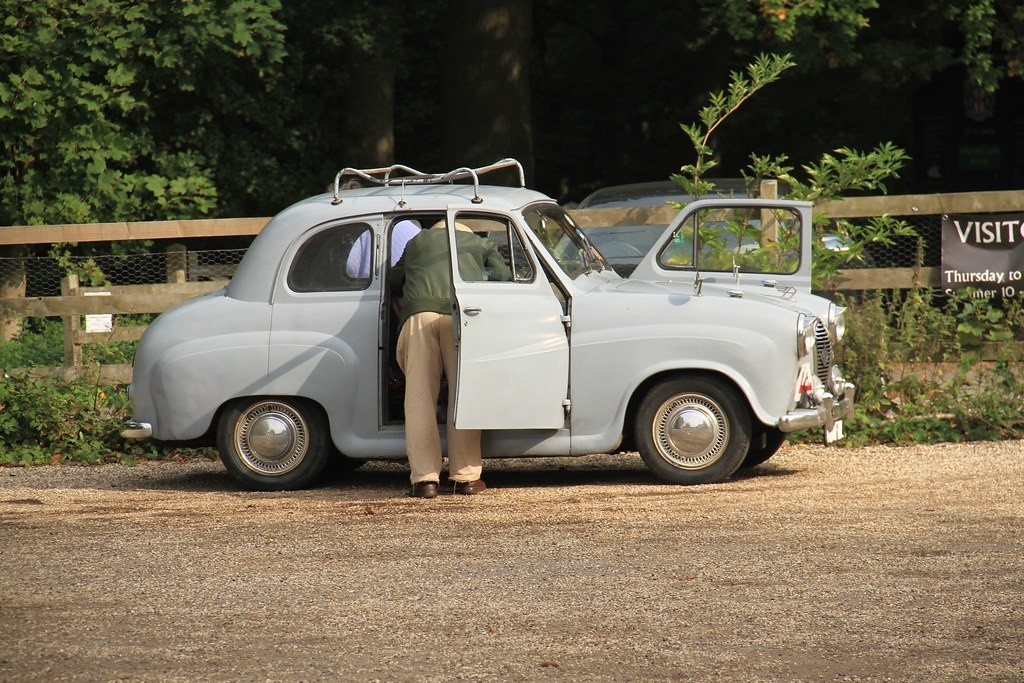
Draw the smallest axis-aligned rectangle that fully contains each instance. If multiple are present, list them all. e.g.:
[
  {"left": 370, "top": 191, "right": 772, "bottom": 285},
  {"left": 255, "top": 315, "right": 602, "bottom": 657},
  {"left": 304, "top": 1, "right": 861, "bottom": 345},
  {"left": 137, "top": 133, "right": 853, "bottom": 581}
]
[
  {"left": 389, "top": 221, "right": 513, "bottom": 499},
  {"left": 346, "top": 219, "right": 421, "bottom": 278}
]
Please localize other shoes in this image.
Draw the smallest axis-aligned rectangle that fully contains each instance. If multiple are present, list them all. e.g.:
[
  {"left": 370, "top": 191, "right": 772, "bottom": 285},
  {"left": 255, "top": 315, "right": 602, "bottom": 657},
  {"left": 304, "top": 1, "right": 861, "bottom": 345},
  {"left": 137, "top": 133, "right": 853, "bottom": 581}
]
[
  {"left": 409, "top": 482, "right": 436, "bottom": 498},
  {"left": 460, "top": 477, "right": 486, "bottom": 495}
]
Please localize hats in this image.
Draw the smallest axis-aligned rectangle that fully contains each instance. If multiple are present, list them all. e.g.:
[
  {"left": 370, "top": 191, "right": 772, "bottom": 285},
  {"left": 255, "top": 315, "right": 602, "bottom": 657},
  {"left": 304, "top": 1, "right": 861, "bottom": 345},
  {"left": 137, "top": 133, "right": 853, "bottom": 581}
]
[{"left": 431, "top": 220, "right": 474, "bottom": 232}]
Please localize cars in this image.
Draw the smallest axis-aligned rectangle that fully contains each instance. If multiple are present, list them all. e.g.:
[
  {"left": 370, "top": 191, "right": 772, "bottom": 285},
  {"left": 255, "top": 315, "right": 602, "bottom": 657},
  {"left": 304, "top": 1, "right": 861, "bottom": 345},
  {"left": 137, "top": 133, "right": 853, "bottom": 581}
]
[{"left": 115, "top": 158, "right": 855, "bottom": 492}]
